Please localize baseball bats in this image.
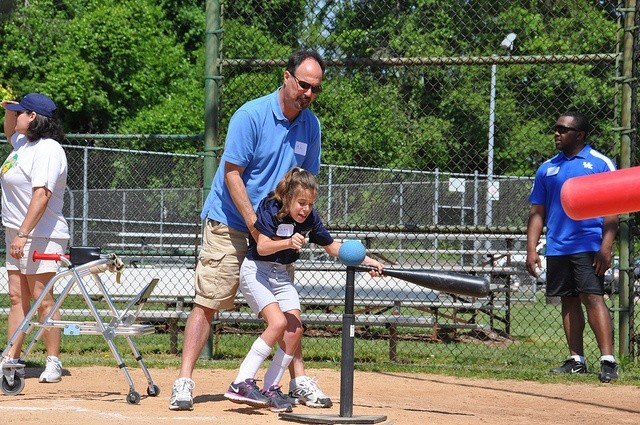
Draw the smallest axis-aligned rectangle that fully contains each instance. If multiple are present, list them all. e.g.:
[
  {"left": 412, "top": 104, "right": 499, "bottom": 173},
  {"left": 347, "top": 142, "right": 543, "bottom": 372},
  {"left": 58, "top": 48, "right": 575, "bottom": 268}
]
[{"left": 345, "top": 264, "right": 489, "bottom": 297}]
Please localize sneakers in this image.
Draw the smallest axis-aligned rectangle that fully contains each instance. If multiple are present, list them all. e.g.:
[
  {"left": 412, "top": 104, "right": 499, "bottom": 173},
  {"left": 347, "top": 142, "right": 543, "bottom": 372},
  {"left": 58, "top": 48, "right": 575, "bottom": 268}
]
[
  {"left": 169, "top": 378, "right": 195, "bottom": 410},
  {"left": 600, "top": 360, "right": 619, "bottom": 383},
  {"left": 39, "top": 356, "right": 62, "bottom": 383},
  {"left": 550, "top": 358, "right": 587, "bottom": 374},
  {"left": 0, "top": 359, "right": 25, "bottom": 377},
  {"left": 259, "top": 385, "right": 293, "bottom": 413},
  {"left": 288, "top": 377, "right": 333, "bottom": 408},
  {"left": 224, "top": 378, "right": 270, "bottom": 407}
]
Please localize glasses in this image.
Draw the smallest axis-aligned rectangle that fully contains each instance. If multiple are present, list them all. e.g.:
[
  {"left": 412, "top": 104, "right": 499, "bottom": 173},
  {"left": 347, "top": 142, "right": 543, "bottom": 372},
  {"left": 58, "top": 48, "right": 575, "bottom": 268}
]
[
  {"left": 287, "top": 69, "right": 322, "bottom": 93},
  {"left": 554, "top": 125, "right": 579, "bottom": 133}
]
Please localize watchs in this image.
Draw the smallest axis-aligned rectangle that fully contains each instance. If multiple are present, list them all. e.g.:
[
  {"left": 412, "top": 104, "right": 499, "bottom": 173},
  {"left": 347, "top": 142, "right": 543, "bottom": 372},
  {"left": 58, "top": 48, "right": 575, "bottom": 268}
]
[{"left": 17, "top": 231, "right": 28, "bottom": 238}]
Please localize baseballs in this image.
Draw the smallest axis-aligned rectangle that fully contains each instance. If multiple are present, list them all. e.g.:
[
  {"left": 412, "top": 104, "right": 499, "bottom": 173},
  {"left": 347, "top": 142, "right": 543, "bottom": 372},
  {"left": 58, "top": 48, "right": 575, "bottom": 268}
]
[{"left": 339, "top": 240, "right": 366, "bottom": 265}]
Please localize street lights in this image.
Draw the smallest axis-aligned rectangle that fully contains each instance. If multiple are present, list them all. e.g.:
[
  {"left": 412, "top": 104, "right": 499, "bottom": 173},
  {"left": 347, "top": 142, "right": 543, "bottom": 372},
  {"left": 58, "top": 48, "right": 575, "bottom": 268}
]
[{"left": 485, "top": 32, "right": 517, "bottom": 290}]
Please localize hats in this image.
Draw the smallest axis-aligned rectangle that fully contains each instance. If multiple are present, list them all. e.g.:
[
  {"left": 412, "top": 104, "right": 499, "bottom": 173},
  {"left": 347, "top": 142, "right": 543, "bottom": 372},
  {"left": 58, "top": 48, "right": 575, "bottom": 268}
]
[{"left": 6, "top": 93, "right": 57, "bottom": 118}]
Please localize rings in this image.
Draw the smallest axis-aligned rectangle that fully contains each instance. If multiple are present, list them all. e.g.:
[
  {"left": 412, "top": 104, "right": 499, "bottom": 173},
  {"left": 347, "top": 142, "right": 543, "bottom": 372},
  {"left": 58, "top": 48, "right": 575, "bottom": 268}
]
[{"left": 14, "top": 248, "right": 21, "bottom": 255}]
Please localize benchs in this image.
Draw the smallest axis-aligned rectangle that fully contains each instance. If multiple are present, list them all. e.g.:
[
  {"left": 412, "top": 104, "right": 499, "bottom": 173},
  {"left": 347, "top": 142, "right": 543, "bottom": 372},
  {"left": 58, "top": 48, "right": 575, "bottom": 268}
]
[
  {"left": 0, "top": 266, "right": 441, "bottom": 367},
  {"left": 392, "top": 266, "right": 516, "bottom": 342}
]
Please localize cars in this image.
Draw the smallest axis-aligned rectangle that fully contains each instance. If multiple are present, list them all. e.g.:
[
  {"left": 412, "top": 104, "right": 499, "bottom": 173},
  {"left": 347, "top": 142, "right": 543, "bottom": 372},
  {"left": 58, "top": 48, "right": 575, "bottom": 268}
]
[{"left": 472, "top": 240, "right": 631, "bottom": 297}]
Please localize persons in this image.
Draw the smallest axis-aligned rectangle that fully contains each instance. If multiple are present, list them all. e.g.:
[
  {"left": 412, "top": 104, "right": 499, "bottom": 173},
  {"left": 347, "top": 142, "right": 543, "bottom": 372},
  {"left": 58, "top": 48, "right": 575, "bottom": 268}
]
[
  {"left": 0, "top": 93, "right": 71, "bottom": 383},
  {"left": 169, "top": 52, "right": 333, "bottom": 412},
  {"left": 526, "top": 111, "right": 619, "bottom": 383},
  {"left": 224, "top": 168, "right": 384, "bottom": 412}
]
